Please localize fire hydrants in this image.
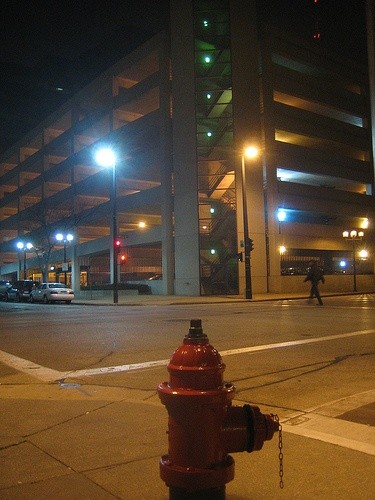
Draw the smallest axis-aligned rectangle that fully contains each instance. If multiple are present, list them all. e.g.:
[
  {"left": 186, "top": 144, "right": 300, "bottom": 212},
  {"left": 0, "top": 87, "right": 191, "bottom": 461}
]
[{"left": 155, "top": 317, "right": 283, "bottom": 500}]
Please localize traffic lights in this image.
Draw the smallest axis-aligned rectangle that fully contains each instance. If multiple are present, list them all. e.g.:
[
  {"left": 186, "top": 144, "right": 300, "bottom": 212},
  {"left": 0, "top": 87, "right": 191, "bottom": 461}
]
[
  {"left": 115, "top": 240, "right": 120, "bottom": 247},
  {"left": 120, "top": 255, "right": 125, "bottom": 262}
]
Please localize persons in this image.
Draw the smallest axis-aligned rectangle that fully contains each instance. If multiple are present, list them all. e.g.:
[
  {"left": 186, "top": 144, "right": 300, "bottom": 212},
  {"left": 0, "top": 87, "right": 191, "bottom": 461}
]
[{"left": 302, "top": 260, "right": 325, "bottom": 305}]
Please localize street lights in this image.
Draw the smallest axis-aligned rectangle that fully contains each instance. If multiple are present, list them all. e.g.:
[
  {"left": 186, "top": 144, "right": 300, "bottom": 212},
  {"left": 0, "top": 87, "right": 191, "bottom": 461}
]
[
  {"left": 54, "top": 233, "right": 74, "bottom": 285},
  {"left": 16, "top": 241, "right": 32, "bottom": 280},
  {"left": 93, "top": 146, "right": 118, "bottom": 303},
  {"left": 240, "top": 145, "right": 260, "bottom": 297},
  {"left": 343, "top": 230, "right": 364, "bottom": 292}
]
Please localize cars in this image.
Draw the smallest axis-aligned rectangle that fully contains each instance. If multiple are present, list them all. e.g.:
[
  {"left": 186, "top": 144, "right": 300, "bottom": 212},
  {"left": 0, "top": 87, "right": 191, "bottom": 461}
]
[
  {"left": 0, "top": 280, "right": 13, "bottom": 300},
  {"left": 29, "top": 282, "right": 75, "bottom": 305}
]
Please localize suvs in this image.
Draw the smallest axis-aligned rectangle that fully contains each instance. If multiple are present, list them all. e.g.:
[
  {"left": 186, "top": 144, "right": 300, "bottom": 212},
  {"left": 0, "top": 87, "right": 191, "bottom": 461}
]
[{"left": 4, "top": 280, "right": 39, "bottom": 303}]
